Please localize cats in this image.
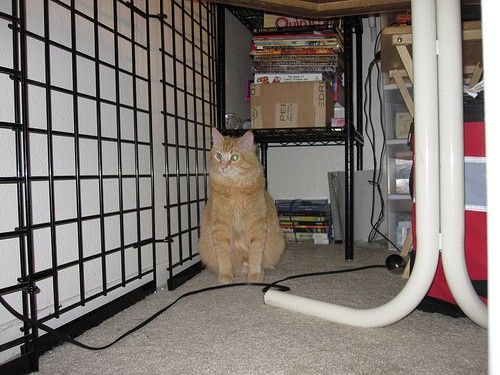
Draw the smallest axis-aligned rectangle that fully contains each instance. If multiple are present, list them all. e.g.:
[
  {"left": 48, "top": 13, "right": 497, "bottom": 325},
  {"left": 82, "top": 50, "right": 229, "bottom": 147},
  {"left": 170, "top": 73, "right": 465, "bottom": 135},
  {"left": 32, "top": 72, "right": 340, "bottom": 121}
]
[{"left": 195, "top": 127, "right": 286, "bottom": 284}]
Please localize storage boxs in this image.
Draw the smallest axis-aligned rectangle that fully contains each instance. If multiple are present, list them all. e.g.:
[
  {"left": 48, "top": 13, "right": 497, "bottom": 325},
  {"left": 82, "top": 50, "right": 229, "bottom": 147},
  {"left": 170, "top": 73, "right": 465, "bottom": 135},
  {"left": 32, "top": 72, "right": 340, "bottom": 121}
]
[{"left": 249, "top": 80, "right": 334, "bottom": 130}]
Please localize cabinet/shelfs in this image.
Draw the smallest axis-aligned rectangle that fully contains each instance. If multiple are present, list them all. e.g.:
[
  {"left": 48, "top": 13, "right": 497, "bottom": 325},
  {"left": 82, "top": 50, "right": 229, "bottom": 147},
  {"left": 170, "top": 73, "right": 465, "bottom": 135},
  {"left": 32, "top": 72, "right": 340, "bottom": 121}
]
[
  {"left": 217, "top": 2, "right": 364, "bottom": 262},
  {"left": 381, "top": 21, "right": 484, "bottom": 253}
]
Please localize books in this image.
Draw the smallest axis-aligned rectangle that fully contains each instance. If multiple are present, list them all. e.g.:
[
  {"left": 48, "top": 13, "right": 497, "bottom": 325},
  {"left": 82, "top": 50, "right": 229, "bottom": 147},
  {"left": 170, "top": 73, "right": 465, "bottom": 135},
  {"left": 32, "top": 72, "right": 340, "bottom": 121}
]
[
  {"left": 248, "top": 25, "right": 346, "bottom": 75},
  {"left": 271, "top": 199, "right": 334, "bottom": 244}
]
